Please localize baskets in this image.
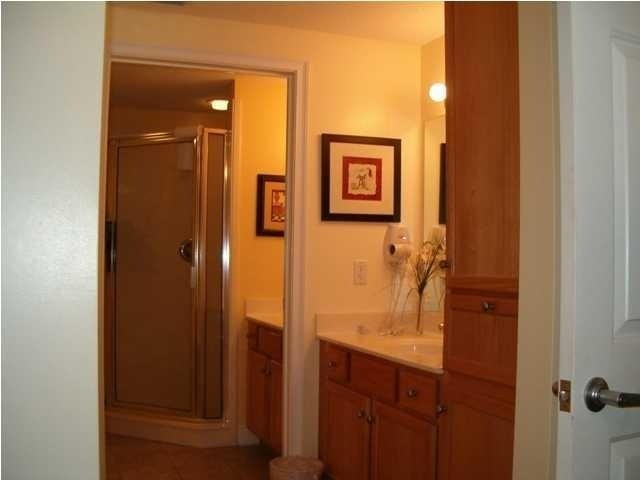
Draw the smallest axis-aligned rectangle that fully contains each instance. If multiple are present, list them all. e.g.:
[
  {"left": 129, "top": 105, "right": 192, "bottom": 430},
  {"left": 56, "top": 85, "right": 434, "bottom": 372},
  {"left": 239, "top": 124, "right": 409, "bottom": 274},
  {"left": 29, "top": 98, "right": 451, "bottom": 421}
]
[{"left": 268, "top": 455, "right": 324, "bottom": 479}]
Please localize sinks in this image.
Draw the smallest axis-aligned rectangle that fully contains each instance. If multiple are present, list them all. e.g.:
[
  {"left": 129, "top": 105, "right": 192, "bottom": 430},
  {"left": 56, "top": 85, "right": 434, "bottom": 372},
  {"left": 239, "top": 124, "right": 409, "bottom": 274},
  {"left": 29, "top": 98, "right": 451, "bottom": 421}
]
[{"left": 394, "top": 339, "right": 443, "bottom": 357}]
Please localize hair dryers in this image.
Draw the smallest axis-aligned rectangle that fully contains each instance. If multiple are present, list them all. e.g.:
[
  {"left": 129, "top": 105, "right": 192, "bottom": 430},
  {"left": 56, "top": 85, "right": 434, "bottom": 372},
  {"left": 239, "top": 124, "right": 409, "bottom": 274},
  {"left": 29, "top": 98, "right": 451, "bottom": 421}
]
[{"left": 387, "top": 243, "right": 413, "bottom": 259}]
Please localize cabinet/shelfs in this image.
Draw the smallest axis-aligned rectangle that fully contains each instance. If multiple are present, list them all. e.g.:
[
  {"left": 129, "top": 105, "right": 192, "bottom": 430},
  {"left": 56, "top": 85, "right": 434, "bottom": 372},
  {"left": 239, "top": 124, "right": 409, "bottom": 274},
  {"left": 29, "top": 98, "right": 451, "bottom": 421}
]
[
  {"left": 320, "top": 338, "right": 440, "bottom": 480},
  {"left": 435, "top": 2, "right": 521, "bottom": 480},
  {"left": 243, "top": 317, "right": 283, "bottom": 455}
]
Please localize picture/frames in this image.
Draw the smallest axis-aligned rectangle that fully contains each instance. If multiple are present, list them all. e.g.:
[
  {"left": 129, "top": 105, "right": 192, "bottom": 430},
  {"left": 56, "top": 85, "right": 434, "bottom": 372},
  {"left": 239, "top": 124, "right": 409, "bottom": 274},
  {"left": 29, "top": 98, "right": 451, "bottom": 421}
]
[
  {"left": 319, "top": 132, "right": 404, "bottom": 225},
  {"left": 254, "top": 173, "right": 286, "bottom": 238}
]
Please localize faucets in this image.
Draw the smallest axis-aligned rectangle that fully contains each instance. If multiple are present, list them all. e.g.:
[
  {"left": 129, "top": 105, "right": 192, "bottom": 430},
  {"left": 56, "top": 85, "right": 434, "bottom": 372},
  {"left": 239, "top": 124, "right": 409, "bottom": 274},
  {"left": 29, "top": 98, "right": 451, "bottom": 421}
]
[{"left": 438, "top": 322, "right": 444, "bottom": 330}]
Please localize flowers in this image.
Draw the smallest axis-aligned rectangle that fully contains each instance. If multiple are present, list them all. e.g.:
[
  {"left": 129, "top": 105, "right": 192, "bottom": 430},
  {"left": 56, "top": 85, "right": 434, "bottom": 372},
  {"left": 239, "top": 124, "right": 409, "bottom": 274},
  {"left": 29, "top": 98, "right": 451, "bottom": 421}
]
[{"left": 401, "top": 224, "right": 446, "bottom": 333}]
